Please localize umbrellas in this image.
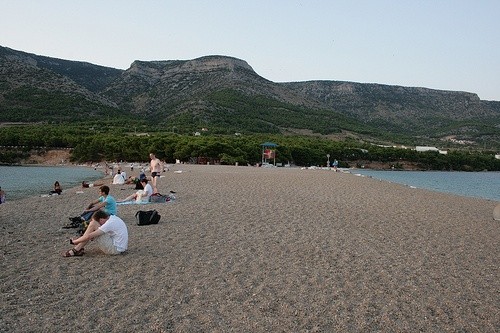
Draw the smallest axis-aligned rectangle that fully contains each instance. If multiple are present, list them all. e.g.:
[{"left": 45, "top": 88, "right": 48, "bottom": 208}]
[{"left": 260, "top": 141, "right": 277, "bottom": 147}]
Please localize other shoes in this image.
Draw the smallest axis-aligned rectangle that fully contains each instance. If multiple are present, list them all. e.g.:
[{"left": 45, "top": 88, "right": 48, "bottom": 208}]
[{"left": 69, "top": 216, "right": 85, "bottom": 223}]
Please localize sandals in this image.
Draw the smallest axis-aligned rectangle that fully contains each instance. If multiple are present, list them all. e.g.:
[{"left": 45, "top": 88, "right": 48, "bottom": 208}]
[{"left": 63, "top": 246, "right": 85, "bottom": 257}]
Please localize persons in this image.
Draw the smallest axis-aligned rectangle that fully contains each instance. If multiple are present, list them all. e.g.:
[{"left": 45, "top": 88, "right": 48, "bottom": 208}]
[
  {"left": 332, "top": 159, "right": 338, "bottom": 172},
  {"left": 82, "top": 181, "right": 104, "bottom": 188},
  {"left": 49, "top": 181, "right": 62, "bottom": 195},
  {"left": 62, "top": 210, "right": 128, "bottom": 257},
  {"left": 149, "top": 153, "right": 163, "bottom": 194},
  {"left": 265, "top": 147, "right": 274, "bottom": 159},
  {"left": 117, "top": 178, "right": 153, "bottom": 205},
  {"left": 112, "top": 170, "right": 146, "bottom": 184},
  {"left": 130, "top": 163, "right": 133, "bottom": 171},
  {"left": 0, "top": 186, "right": 7, "bottom": 204},
  {"left": 79, "top": 186, "right": 117, "bottom": 221}
]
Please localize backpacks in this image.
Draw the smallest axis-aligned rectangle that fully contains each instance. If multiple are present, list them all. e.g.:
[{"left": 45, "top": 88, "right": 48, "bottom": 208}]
[
  {"left": 134, "top": 209, "right": 161, "bottom": 226},
  {"left": 150, "top": 193, "right": 170, "bottom": 202}
]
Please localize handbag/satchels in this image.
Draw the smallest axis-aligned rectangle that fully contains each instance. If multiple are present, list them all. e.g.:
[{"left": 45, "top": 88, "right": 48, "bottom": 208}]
[{"left": 135, "top": 178, "right": 144, "bottom": 190}]
